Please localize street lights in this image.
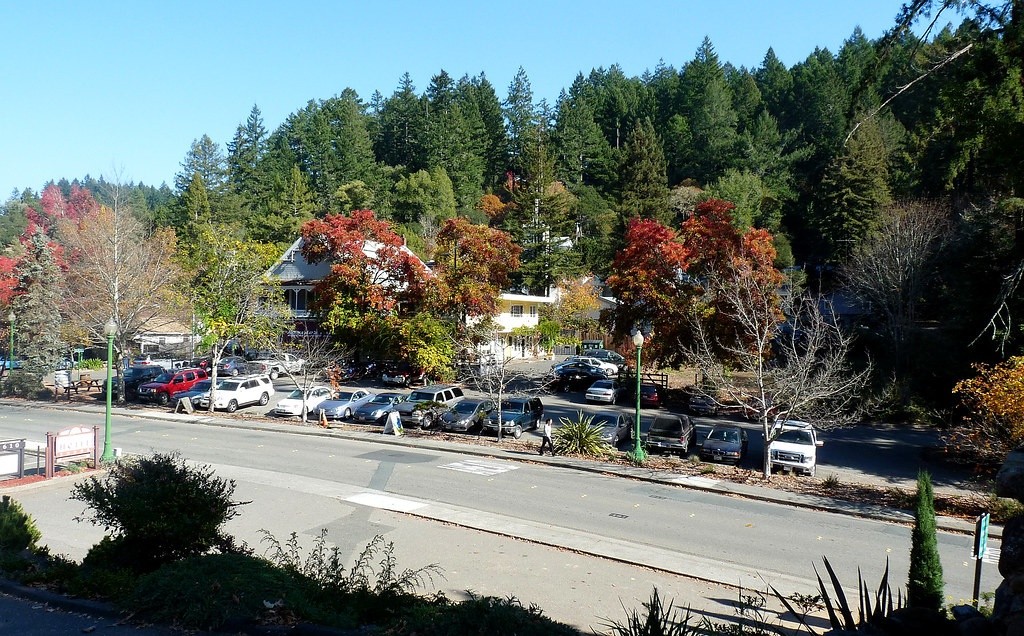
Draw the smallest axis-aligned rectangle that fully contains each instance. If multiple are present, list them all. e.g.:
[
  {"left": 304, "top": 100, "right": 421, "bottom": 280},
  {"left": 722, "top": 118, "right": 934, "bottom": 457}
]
[
  {"left": 628, "top": 330, "right": 645, "bottom": 461},
  {"left": 98, "top": 316, "right": 118, "bottom": 464},
  {"left": 8, "top": 312, "right": 16, "bottom": 377}
]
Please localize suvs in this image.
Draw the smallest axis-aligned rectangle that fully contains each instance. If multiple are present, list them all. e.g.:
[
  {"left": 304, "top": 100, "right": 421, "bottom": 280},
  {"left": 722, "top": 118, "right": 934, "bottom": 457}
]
[
  {"left": 135, "top": 369, "right": 208, "bottom": 405},
  {"left": 101, "top": 364, "right": 168, "bottom": 403},
  {"left": 760, "top": 419, "right": 824, "bottom": 479},
  {"left": 391, "top": 385, "right": 465, "bottom": 429},
  {"left": 480, "top": 397, "right": 543, "bottom": 439}
]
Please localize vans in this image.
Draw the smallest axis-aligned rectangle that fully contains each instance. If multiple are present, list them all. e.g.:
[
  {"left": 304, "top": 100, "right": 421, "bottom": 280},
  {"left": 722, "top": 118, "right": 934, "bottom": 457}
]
[{"left": 645, "top": 414, "right": 697, "bottom": 455}]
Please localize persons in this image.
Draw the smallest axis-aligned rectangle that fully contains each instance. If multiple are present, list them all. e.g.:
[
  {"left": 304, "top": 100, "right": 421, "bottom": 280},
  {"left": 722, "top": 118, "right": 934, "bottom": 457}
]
[{"left": 540, "top": 418, "right": 557, "bottom": 457}]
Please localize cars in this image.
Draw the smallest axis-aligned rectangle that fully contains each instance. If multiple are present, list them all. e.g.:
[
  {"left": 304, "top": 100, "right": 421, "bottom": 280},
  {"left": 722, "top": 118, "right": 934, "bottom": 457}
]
[
  {"left": 585, "top": 411, "right": 634, "bottom": 449},
  {"left": 585, "top": 381, "right": 623, "bottom": 405},
  {"left": 437, "top": 398, "right": 494, "bottom": 433},
  {"left": 700, "top": 425, "right": 750, "bottom": 467},
  {"left": 170, "top": 378, "right": 223, "bottom": 409},
  {"left": 274, "top": 386, "right": 338, "bottom": 418},
  {"left": 352, "top": 392, "right": 407, "bottom": 425},
  {"left": 543, "top": 348, "right": 629, "bottom": 392},
  {"left": 189, "top": 374, "right": 276, "bottom": 413},
  {"left": 130, "top": 353, "right": 165, "bottom": 367},
  {"left": 312, "top": 389, "right": 380, "bottom": 421},
  {"left": 0, "top": 354, "right": 26, "bottom": 371},
  {"left": 687, "top": 396, "right": 719, "bottom": 418},
  {"left": 381, "top": 365, "right": 413, "bottom": 386},
  {"left": 190, "top": 353, "right": 314, "bottom": 379},
  {"left": 633, "top": 381, "right": 662, "bottom": 408}
]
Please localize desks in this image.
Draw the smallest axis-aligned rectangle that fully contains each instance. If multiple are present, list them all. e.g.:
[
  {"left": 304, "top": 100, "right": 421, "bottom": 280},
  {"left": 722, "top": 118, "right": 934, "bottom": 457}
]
[{"left": 71, "top": 378, "right": 102, "bottom": 391}]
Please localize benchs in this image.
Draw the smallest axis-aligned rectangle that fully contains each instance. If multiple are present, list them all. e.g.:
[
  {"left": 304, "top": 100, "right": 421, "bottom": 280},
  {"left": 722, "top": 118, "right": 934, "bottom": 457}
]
[{"left": 64, "top": 385, "right": 103, "bottom": 394}]
